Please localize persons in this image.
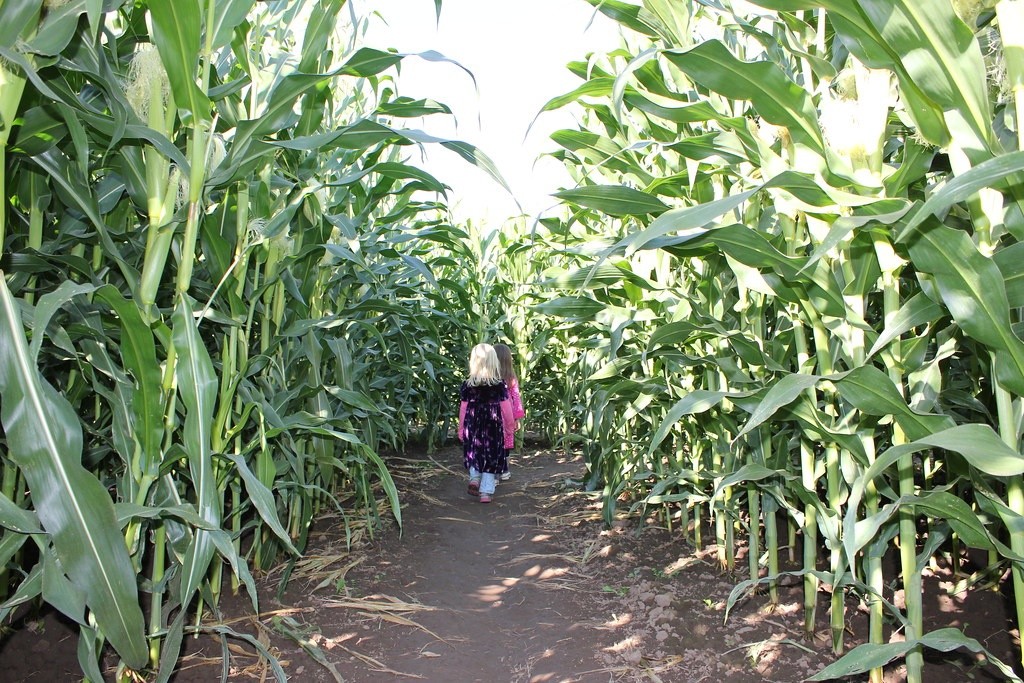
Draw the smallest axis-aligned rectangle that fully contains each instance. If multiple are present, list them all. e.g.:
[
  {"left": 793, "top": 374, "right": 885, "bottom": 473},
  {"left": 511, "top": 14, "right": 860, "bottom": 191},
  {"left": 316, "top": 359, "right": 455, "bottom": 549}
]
[
  {"left": 459, "top": 342, "right": 510, "bottom": 504},
  {"left": 458, "top": 344, "right": 526, "bottom": 487}
]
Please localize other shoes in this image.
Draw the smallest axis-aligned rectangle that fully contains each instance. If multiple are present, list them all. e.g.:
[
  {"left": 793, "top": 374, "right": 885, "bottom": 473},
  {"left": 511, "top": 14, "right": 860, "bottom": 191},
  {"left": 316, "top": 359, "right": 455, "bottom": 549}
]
[
  {"left": 494, "top": 479, "right": 500, "bottom": 486},
  {"left": 467, "top": 477, "right": 480, "bottom": 495},
  {"left": 500, "top": 471, "right": 511, "bottom": 480},
  {"left": 480, "top": 493, "right": 492, "bottom": 502}
]
